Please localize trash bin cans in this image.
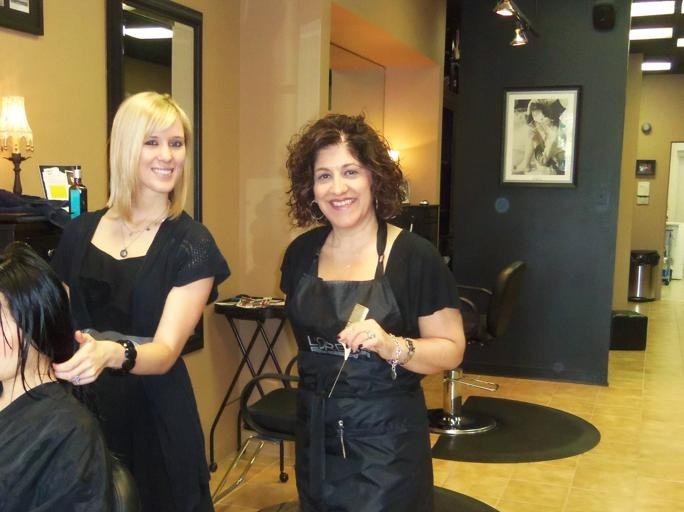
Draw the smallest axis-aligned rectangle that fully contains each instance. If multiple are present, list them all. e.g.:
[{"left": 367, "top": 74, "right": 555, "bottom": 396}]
[{"left": 628, "top": 250, "right": 660, "bottom": 302}]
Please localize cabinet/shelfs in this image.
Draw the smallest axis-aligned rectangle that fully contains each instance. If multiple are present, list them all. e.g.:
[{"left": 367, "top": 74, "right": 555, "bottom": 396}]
[{"left": 399, "top": 203, "right": 440, "bottom": 251}]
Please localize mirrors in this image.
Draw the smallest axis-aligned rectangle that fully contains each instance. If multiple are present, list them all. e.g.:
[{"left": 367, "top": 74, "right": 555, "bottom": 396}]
[{"left": 105, "top": 0, "right": 205, "bottom": 357}]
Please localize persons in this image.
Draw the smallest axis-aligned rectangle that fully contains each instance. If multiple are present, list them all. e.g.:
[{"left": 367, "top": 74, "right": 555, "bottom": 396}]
[
  {"left": 49, "top": 92, "right": 232, "bottom": 512},
  {"left": 281, "top": 112, "right": 468, "bottom": 512},
  {"left": 0, "top": 242, "right": 112, "bottom": 512}
]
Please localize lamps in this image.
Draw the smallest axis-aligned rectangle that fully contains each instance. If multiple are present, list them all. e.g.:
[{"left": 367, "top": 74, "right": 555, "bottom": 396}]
[
  {"left": 0, "top": 95, "right": 35, "bottom": 195},
  {"left": 495, "top": 0, "right": 529, "bottom": 46}
]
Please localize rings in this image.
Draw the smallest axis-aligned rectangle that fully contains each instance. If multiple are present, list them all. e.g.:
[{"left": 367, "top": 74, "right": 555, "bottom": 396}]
[
  {"left": 366, "top": 330, "right": 377, "bottom": 339},
  {"left": 74, "top": 375, "right": 83, "bottom": 386}
]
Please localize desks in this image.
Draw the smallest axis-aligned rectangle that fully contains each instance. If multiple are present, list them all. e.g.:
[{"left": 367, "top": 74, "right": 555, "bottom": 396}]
[{"left": 205, "top": 295, "right": 296, "bottom": 481}]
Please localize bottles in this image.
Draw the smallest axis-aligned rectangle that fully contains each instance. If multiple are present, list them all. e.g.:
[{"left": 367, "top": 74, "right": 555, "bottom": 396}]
[{"left": 68, "top": 170, "right": 88, "bottom": 219}]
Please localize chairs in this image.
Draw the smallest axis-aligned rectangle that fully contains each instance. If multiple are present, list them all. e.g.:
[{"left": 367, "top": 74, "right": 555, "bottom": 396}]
[
  {"left": 214, "top": 357, "right": 300, "bottom": 503},
  {"left": 106, "top": 451, "right": 143, "bottom": 511},
  {"left": 426, "top": 260, "right": 528, "bottom": 443}
]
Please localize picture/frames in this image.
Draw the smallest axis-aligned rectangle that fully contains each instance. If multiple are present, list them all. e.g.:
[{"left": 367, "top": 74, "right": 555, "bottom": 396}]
[
  {"left": 498, "top": 84, "right": 583, "bottom": 190},
  {"left": 42, "top": 165, "right": 75, "bottom": 202},
  {"left": 635, "top": 159, "right": 657, "bottom": 176},
  {"left": 0, "top": 0, "right": 45, "bottom": 36}
]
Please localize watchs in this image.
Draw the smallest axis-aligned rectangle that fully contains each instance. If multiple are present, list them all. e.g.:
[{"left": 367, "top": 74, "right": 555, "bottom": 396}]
[{"left": 399, "top": 337, "right": 415, "bottom": 367}]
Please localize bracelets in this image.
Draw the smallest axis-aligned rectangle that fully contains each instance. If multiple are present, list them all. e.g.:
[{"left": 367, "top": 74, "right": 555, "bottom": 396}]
[
  {"left": 387, "top": 333, "right": 401, "bottom": 380},
  {"left": 109, "top": 339, "right": 137, "bottom": 378}
]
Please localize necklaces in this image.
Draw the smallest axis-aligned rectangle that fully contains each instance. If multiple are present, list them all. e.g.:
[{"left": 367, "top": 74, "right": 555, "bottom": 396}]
[
  {"left": 343, "top": 264, "right": 353, "bottom": 267},
  {"left": 119, "top": 204, "right": 167, "bottom": 257}
]
